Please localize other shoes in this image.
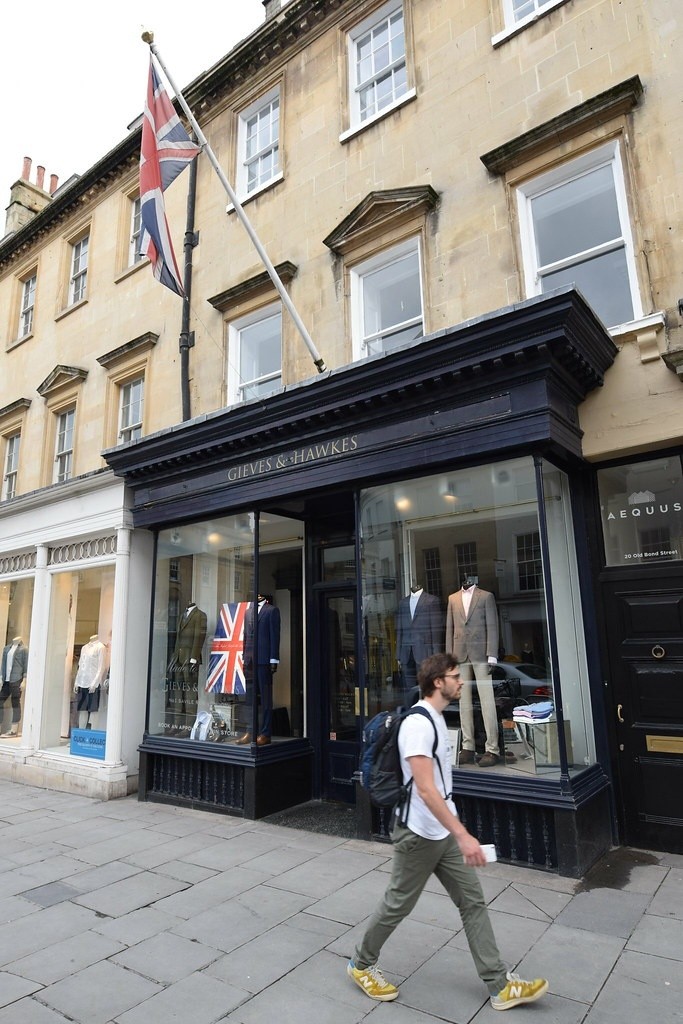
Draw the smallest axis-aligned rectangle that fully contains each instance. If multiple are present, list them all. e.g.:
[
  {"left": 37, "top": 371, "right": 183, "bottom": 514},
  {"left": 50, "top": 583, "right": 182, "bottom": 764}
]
[
  {"left": 1, "top": 731, "right": 17, "bottom": 737},
  {"left": 458, "top": 749, "right": 475, "bottom": 764},
  {"left": 235, "top": 733, "right": 252, "bottom": 744},
  {"left": 478, "top": 751, "right": 499, "bottom": 766},
  {"left": 257, "top": 735, "right": 271, "bottom": 745}
]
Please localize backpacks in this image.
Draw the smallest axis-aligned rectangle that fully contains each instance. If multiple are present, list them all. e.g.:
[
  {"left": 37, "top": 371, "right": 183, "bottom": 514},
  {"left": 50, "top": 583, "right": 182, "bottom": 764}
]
[{"left": 356, "top": 704, "right": 439, "bottom": 807}]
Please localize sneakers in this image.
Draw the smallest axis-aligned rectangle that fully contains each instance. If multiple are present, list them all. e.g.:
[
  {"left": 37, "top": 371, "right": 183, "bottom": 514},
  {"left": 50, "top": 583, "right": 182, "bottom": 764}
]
[
  {"left": 490, "top": 971, "right": 549, "bottom": 1011},
  {"left": 346, "top": 959, "right": 399, "bottom": 1001}
]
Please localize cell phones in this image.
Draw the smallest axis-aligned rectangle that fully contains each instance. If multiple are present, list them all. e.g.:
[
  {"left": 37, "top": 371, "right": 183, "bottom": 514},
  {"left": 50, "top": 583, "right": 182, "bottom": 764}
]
[{"left": 462, "top": 844, "right": 497, "bottom": 864}]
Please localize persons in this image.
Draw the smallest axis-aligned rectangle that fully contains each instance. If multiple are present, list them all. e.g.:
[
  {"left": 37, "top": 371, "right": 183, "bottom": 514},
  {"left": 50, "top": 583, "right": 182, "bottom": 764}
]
[
  {"left": 236, "top": 597, "right": 281, "bottom": 746},
  {"left": 74, "top": 635, "right": 109, "bottom": 730},
  {"left": 397, "top": 584, "right": 443, "bottom": 706},
  {"left": 0, "top": 638, "right": 28, "bottom": 735},
  {"left": 348, "top": 654, "right": 549, "bottom": 1011},
  {"left": 164, "top": 603, "right": 207, "bottom": 737},
  {"left": 445, "top": 581, "right": 500, "bottom": 766}
]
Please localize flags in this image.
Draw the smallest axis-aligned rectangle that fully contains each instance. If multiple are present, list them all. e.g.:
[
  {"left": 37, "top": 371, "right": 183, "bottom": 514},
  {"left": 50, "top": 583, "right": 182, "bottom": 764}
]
[
  {"left": 205, "top": 601, "right": 250, "bottom": 694},
  {"left": 138, "top": 54, "right": 202, "bottom": 298}
]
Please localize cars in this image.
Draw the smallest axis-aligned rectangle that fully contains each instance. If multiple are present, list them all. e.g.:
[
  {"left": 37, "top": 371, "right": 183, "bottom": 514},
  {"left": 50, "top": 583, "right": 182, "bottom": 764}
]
[{"left": 405, "top": 662, "right": 556, "bottom": 720}]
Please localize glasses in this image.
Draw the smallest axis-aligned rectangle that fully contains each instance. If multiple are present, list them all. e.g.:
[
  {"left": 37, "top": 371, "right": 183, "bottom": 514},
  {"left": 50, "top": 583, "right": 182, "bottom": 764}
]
[{"left": 444, "top": 673, "right": 460, "bottom": 680}]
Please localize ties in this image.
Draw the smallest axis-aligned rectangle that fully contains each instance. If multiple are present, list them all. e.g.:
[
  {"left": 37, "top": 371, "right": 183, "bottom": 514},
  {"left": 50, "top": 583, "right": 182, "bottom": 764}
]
[
  {"left": 193, "top": 721, "right": 203, "bottom": 740},
  {"left": 181, "top": 608, "right": 189, "bottom": 627}
]
